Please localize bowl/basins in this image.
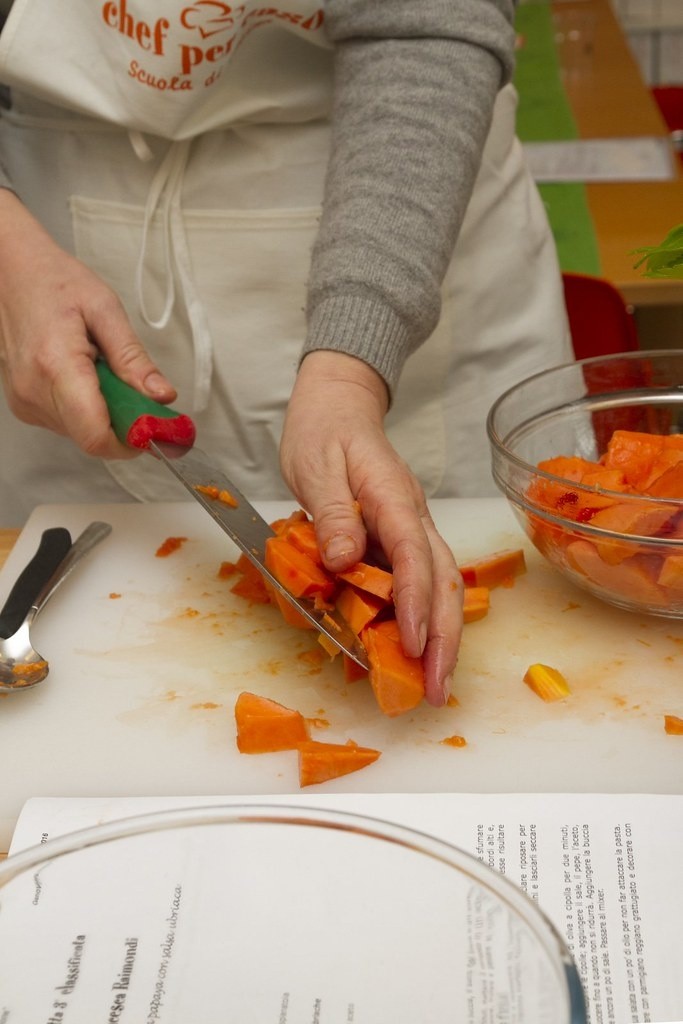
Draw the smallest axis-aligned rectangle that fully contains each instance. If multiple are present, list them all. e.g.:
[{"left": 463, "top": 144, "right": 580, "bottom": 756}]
[
  {"left": 0, "top": 804, "right": 584, "bottom": 1024},
  {"left": 485, "top": 351, "right": 683, "bottom": 620}
]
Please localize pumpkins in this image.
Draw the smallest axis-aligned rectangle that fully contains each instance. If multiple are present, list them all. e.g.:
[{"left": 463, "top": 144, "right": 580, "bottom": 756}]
[
  {"left": 222, "top": 511, "right": 576, "bottom": 787},
  {"left": 524, "top": 428, "right": 683, "bottom": 612}
]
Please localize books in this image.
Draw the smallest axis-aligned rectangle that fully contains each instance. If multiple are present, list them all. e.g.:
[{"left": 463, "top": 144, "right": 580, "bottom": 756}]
[{"left": 0, "top": 793, "right": 683, "bottom": 1024}]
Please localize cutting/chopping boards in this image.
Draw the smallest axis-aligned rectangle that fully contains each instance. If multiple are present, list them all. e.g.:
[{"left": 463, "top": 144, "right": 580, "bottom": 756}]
[{"left": 0, "top": 500, "right": 683, "bottom": 854}]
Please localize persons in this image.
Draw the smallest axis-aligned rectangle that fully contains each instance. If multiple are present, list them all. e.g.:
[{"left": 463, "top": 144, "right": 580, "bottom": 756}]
[{"left": 0, "top": 0, "right": 599, "bottom": 711}]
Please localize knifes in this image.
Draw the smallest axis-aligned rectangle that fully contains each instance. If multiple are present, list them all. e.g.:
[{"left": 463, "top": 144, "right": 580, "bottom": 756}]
[{"left": 95, "top": 360, "right": 371, "bottom": 671}]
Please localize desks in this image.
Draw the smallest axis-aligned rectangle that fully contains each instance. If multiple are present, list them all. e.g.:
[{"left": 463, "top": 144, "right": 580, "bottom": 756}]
[{"left": 512, "top": 1, "right": 682, "bottom": 310}]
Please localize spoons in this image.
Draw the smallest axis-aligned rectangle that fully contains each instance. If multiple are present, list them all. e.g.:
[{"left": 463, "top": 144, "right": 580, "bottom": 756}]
[{"left": 0, "top": 521, "right": 112, "bottom": 690}]
